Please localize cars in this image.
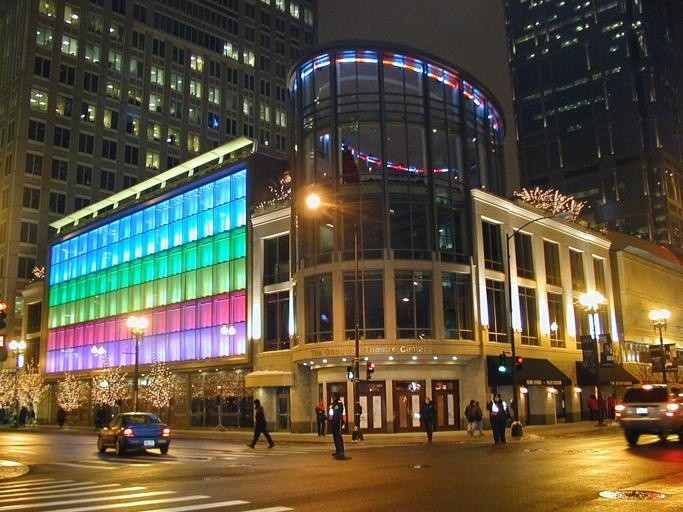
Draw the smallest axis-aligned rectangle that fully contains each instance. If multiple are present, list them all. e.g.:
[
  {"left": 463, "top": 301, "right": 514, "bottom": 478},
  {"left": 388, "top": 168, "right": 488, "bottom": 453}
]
[{"left": 95, "top": 412, "right": 171, "bottom": 457}]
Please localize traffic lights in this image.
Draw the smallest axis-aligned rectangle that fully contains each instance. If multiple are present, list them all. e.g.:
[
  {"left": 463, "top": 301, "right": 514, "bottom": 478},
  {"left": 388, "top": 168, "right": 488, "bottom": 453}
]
[
  {"left": 345, "top": 365, "right": 355, "bottom": 380},
  {"left": 496, "top": 355, "right": 507, "bottom": 377},
  {"left": 365, "top": 361, "right": 375, "bottom": 381},
  {"left": 515, "top": 354, "right": 523, "bottom": 371},
  {"left": 0, "top": 300, "right": 7, "bottom": 330}
]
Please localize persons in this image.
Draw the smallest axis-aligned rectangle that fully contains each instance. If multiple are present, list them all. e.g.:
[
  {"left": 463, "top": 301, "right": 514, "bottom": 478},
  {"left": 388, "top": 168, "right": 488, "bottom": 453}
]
[
  {"left": 587, "top": 392, "right": 618, "bottom": 421},
  {"left": 248, "top": 399, "right": 275, "bottom": 449},
  {"left": 330, "top": 392, "right": 344, "bottom": 458},
  {"left": 470, "top": 400, "right": 485, "bottom": 437},
  {"left": 420, "top": 397, "right": 436, "bottom": 442},
  {"left": 465, "top": 400, "right": 475, "bottom": 434},
  {"left": 315, "top": 401, "right": 326, "bottom": 437},
  {"left": 486, "top": 393, "right": 511, "bottom": 446}
]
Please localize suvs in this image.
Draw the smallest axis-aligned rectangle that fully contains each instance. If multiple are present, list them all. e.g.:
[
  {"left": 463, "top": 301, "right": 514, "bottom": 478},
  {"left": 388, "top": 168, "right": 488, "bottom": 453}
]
[{"left": 612, "top": 383, "right": 682, "bottom": 445}]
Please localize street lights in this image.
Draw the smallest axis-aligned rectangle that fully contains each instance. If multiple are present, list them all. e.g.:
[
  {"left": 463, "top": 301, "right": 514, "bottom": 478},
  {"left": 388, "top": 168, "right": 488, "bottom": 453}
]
[
  {"left": 504, "top": 209, "right": 578, "bottom": 440},
  {"left": 550, "top": 321, "right": 558, "bottom": 335},
  {"left": 306, "top": 194, "right": 362, "bottom": 442},
  {"left": 647, "top": 308, "right": 669, "bottom": 383},
  {"left": 126, "top": 315, "right": 148, "bottom": 412},
  {"left": 9, "top": 341, "right": 27, "bottom": 428},
  {"left": 580, "top": 290, "right": 608, "bottom": 429}
]
